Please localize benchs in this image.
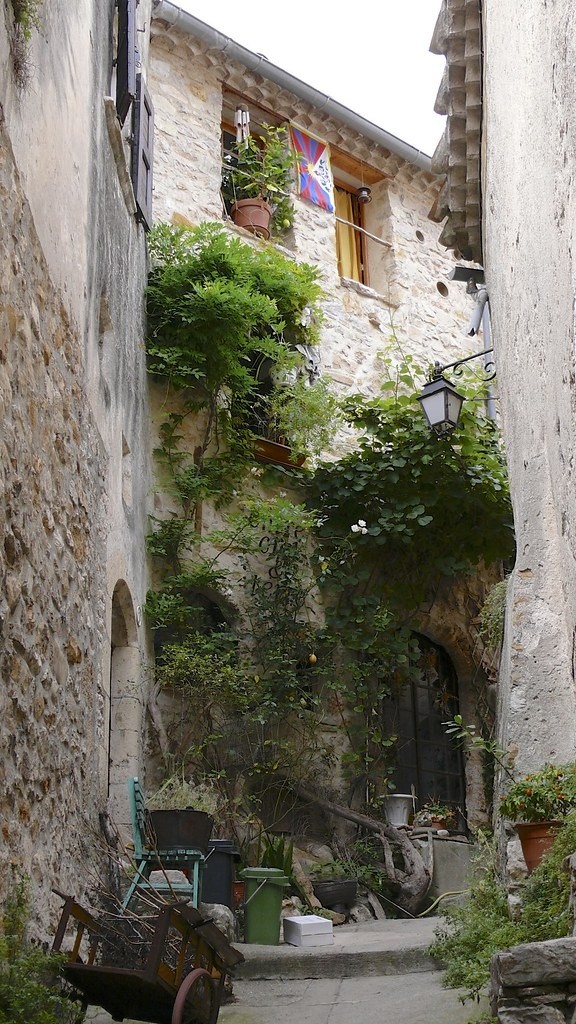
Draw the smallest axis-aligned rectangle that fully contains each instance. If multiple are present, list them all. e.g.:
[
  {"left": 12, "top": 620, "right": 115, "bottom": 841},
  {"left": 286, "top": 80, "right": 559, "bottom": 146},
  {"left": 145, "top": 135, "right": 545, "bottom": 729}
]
[{"left": 119, "top": 776, "right": 208, "bottom": 910}]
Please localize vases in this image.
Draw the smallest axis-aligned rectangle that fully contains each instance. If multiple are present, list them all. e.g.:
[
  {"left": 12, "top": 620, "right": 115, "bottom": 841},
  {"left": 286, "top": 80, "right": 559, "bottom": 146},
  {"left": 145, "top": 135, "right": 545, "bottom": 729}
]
[{"left": 379, "top": 793, "right": 419, "bottom": 828}]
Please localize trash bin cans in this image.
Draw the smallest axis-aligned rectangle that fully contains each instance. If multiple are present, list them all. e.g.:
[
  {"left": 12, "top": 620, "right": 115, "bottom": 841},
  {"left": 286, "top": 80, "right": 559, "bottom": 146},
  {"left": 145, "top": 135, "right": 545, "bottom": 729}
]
[
  {"left": 238, "top": 867, "right": 291, "bottom": 946},
  {"left": 201, "top": 838, "right": 239, "bottom": 907}
]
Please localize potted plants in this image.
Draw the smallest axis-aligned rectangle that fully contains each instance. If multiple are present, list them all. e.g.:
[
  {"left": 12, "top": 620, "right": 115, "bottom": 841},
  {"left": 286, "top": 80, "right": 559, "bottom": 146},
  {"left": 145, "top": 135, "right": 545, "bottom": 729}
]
[
  {"left": 146, "top": 774, "right": 228, "bottom": 854},
  {"left": 250, "top": 429, "right": 312, "bottom": 467},
  {"left": 220, "top": 120, "right": 298, "bottom": 244},
  {"left": 232, "top": 829, "right": 296, "bottom": 909},
  {"left": 501, "top": 763, "right": 576, "bottom": 873},
  {"left": 416, "top": 792, "right": 457, "bottom": 830},
  {"left": 307, "top": 857, "right": 375, "bottom": 906}
]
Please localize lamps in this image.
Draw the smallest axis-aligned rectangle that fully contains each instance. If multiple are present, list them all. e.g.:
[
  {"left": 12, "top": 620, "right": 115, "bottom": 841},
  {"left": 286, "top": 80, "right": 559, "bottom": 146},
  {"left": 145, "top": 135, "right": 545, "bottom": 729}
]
[
  {"left": 417, "top": 347, "right": 503, "bottom": 440},
  {"left": 357, "top": 159, "right": 372, "bottom": 204}
]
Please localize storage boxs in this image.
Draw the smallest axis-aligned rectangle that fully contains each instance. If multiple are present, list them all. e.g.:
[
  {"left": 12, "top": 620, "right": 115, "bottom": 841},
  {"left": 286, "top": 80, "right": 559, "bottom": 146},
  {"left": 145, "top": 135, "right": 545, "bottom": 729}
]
[{"left": 284, "top": 915, "right": 334, "bottom": 948}]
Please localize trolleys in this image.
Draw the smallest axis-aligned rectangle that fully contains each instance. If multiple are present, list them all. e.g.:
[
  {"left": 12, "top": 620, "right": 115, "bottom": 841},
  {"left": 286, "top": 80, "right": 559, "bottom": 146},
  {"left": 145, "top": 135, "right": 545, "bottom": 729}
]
[{"left": 38, "top": 888, "right": 247, "bottom": 1024}]
